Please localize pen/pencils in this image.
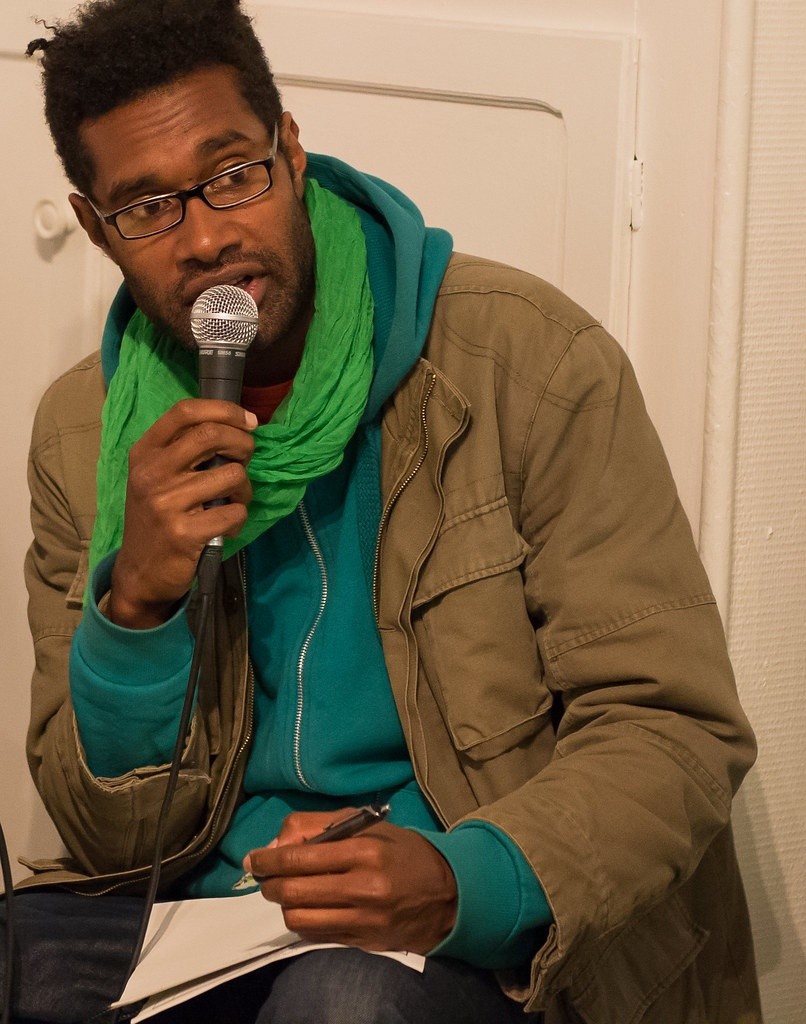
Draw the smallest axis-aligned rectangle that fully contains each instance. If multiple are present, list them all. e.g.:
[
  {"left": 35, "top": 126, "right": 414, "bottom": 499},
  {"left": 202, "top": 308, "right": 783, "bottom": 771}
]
[{"left": 231, "top": 803, "right": 391, "bottom": 892}]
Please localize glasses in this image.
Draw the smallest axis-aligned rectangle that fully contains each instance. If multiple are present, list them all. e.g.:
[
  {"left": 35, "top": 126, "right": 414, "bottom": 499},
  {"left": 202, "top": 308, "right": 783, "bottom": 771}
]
[{"left": 78, "top": 118, "right": 280, "bottom": 241}]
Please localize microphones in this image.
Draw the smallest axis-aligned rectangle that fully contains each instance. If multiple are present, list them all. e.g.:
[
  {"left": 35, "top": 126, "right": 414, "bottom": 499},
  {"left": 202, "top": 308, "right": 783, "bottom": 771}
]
[{"left": 188, "top": 285, "right": 258, "bottom": 594}]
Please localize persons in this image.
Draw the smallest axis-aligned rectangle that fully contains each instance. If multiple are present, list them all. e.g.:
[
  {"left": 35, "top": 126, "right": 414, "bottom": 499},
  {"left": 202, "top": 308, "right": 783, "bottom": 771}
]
[{"left": 0, "top": 2, "right": 764, "bottom": 1023}]
